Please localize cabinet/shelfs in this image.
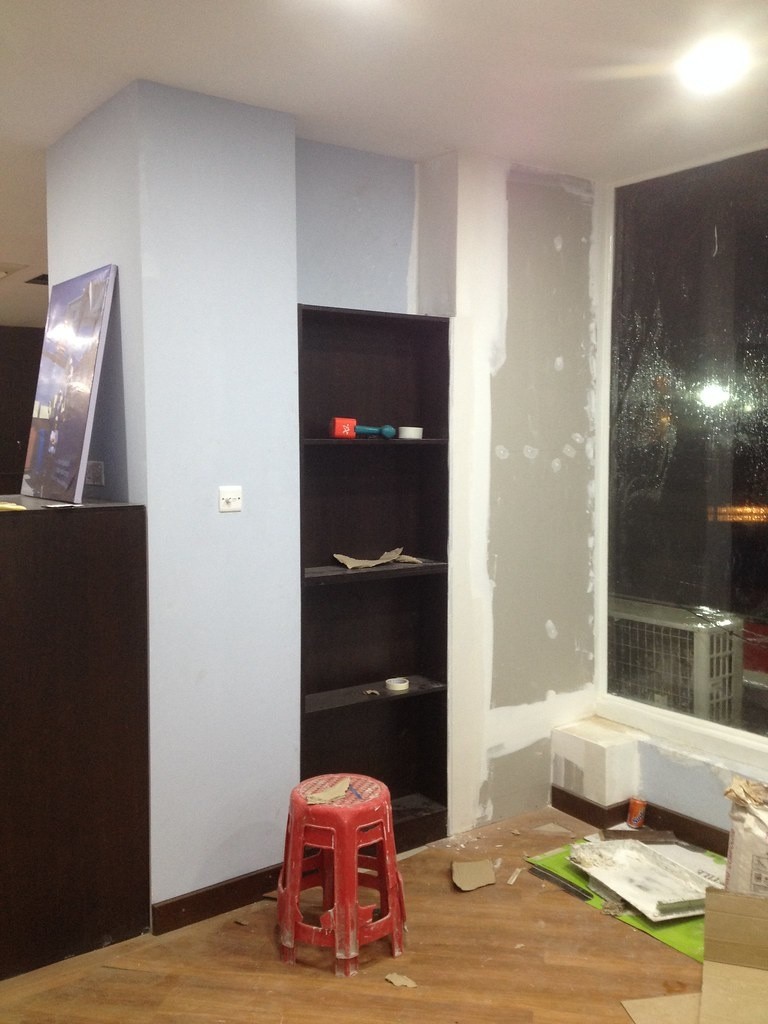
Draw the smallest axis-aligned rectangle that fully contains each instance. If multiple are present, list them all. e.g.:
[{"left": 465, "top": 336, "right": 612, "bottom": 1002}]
[
  {"left": 296, "top": 302, "right": 450, "bottom": 880},
  {"left": 0, "top": 493, "right": 152, "bottom": 980}
]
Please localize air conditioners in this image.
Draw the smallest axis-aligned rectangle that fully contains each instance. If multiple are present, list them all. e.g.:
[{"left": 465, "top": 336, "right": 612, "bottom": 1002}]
[{"left": 608, "top": 594, "right": 743, "bottom": 729}]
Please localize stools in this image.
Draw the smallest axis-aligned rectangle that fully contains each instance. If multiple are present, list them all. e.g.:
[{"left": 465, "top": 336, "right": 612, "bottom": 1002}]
[{"left": 278, "top": 773, "right": 407, "bottom": 976}]
